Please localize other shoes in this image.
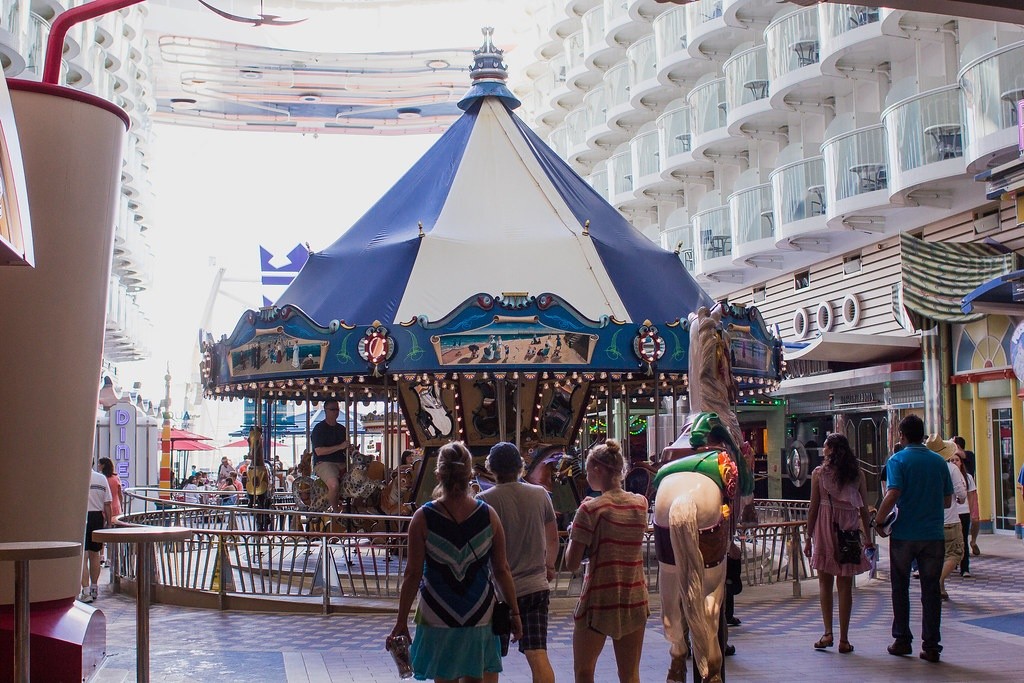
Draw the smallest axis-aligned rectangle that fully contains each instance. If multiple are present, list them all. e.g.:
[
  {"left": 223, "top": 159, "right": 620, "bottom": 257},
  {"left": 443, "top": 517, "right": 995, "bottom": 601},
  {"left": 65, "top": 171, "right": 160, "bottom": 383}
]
[
  {"left": 726, "top": 617, "right": 741, "bottom": 626},
  {"left": 82, "top": 586, "right": 98, "bottom": 604},
  {"left": 919, "top": 651, "right": 940, "bottom": 663},
  {"left": 913, "top": 570, "right": 919, "bottom": 579},
  {"left": 887, "top": 639, "right": 913, "bottom": 655},
  {"left": 105, "top": 559, "right": 112, "bottom": 568},
  {"left": 970, "top": 541, "right": 980, "bottom": 554},
  {"left": 941, "top": 592, "right": 949, "bottom": 602},
  {"left": 725, "top": 643, "right": 735, "bottom": 657},
  {"left": 963, "top": 572, "right": 971, "bottom": 578},
  {"left": 100, "top": 555, "right": 105, "bottom": 565}
]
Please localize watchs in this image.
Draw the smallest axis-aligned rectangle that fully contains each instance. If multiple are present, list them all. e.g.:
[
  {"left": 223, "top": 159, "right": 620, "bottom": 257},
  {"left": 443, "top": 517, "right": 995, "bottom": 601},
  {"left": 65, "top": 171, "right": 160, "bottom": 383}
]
[{"left": 805, "top": 533, "right": 812, "bottom": 539}]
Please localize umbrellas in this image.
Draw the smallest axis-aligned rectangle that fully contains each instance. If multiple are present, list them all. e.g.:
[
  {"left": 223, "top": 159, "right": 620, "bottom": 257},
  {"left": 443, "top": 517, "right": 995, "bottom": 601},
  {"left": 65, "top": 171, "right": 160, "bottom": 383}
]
[{"left": 156, "top": 428, "right": 291, "bottom": 485}]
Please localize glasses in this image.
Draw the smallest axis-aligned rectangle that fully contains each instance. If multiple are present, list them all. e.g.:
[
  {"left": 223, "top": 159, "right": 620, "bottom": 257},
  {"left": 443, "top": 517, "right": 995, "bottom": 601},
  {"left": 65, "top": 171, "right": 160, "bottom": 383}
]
[{"left": 324, "top": 408, "right": 339, "bottom": 412}]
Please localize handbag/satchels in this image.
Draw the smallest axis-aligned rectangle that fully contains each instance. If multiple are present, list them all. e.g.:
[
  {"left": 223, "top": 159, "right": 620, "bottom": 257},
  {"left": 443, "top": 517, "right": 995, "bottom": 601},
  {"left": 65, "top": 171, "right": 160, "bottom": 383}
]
[
  {"left": 491, "top": 600, "right": 511, "bottom": 635},
  {"left": 727, "top": 552, "right": 743, "bottom": 596},
  {"left": 836, "top": 529, "right": 863, "bottom": 564}
]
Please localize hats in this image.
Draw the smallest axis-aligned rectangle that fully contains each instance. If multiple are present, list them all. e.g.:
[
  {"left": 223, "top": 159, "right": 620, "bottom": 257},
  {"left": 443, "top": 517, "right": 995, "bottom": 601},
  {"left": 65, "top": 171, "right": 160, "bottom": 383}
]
[
  {"left": 221, "top": 457, "right": 228, "bottom": 461},
  {"left": 923, "top": 433, "right": 957, "bottom": 460},
  {"left": 489, "top": 441, "right": 522, "bottom": 471}
]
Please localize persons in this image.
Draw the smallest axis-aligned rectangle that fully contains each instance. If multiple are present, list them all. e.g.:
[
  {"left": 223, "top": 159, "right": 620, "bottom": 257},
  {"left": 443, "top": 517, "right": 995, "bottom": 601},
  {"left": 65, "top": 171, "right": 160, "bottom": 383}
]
[
  {"left": 875, "top": 414, "right": 954, "bottom": 663},
  {"left": 474, "top": 442, "right": 560, "bottom": 683},
  {"left": 77, "top": 455, "right": 301, "bottom": 604},
  {"left": 400, "top": 450, "right": 415, "bottom": 464},
  {"left": 879, "top": 434, "right": 980, "bottom": 602},
  {"left": 565, "top": 439, "right": 651, "bottom": 683},
  {"left": 311, "top": 398, "right": 360, "bottom": 520},
  {"left": 804, "top": 433, "right": 877, "bottom": 653},
  {"left": 386, "top": 443, "right": 524, "bottom": 683}
]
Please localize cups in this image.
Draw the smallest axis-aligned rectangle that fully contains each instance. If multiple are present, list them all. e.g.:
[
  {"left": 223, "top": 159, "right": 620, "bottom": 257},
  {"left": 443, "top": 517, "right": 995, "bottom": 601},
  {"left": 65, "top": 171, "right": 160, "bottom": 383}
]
[{"left": 389, "top": 636, "right": 415, "bottom": 679}]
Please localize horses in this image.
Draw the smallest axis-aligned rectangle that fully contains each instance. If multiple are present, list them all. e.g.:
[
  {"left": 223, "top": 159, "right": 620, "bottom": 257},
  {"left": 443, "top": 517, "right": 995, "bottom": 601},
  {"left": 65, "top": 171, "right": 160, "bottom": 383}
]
[
  {"left": 653, "top": 301, "right": 756, "bottom": 683},
  {"left": 245, "top": 424, "right": 658, "bottom": 547}
]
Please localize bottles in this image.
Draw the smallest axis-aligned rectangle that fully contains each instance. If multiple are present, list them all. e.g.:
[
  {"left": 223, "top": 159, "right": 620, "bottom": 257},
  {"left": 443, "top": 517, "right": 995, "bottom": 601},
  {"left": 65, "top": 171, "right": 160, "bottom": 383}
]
[{"left": 567, "top": 522, "right": 591, "bottom": 565}]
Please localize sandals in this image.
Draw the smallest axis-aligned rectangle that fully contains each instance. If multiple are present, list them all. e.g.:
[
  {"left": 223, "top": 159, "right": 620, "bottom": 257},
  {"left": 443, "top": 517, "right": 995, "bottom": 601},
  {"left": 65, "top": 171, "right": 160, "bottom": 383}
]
[
  {"left": 814, "top": 632, "right": 833, "bottom": 649},
  {"left": 839, "top": 640, "right": 854, "bottom": 653}
]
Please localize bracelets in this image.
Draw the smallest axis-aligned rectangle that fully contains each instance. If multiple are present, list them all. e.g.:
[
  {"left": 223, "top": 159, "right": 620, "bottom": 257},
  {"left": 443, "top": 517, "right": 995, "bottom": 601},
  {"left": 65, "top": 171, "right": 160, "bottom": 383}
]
[
  {"left": 509, "top": 613, "right": 520, "bottom": 616},
  {"left": 876, "top": 522, "right": 885, "bottom": 528}
]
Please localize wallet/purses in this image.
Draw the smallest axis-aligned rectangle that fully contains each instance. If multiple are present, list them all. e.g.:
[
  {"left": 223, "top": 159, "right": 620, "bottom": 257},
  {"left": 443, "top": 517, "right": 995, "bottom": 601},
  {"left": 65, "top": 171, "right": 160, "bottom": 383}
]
[{"left": 884, "top": 511, "right": 896, "bottom": 527}]
